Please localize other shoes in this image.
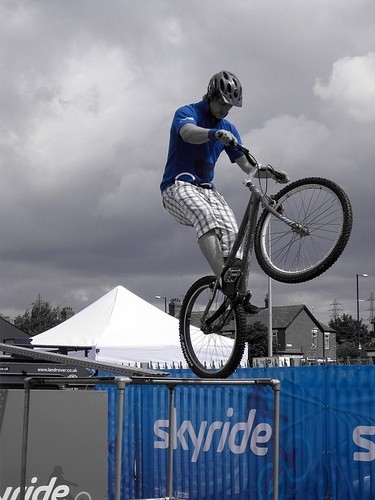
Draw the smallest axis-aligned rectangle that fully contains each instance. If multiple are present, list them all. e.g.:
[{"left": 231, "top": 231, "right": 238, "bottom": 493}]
[
  {"left": 218, "top": 258, "right": 243, "bottom": 298},
  {"left": 244, "top": 302, "right": 260, "bottom": 315}
]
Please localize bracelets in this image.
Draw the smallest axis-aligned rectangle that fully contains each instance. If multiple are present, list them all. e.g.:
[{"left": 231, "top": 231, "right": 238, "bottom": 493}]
[{"left": 208, "top": 128, "right": 218, "bottom": 141}]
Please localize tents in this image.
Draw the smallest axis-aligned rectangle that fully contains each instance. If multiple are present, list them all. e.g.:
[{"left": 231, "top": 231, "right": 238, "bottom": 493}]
[{"left": 28, "top": 285, "right": 248, "bottom": 368}]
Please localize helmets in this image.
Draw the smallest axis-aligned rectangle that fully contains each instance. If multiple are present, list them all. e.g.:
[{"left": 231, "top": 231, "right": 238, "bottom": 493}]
[{"left": 207, "top": 70, "right": 243, "bottom": 107}]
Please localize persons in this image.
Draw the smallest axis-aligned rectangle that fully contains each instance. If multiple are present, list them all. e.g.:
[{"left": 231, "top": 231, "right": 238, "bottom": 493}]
[{"left": 159, "top": 71, "right": 289, "bottom": 315}]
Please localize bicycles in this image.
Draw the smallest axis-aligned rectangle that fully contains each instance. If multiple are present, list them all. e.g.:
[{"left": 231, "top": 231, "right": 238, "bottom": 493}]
[{"left": 175, "top": 132, "right": 353, "bottom": 380}]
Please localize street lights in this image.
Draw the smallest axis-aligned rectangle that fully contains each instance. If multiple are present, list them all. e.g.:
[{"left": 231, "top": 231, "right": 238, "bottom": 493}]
[
  {"left": 353, "top": 269, "right": 368, "bottom": 320},
  {"left": 155, "top": 294, "right": 168, "bottom": 313}
]
